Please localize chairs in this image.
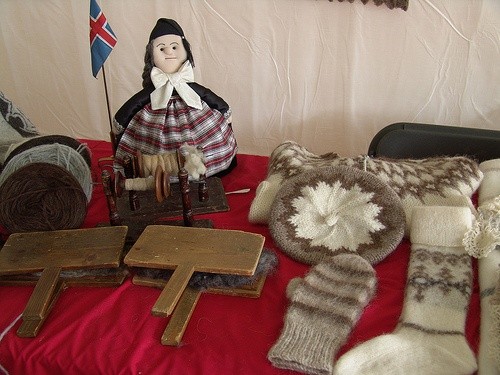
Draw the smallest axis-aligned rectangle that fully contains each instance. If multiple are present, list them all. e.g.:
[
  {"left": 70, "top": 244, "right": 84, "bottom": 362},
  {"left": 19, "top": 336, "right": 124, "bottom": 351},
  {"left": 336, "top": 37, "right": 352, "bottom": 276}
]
[{"left": 368, "top": 122, "right": 500, "bottom": 163}]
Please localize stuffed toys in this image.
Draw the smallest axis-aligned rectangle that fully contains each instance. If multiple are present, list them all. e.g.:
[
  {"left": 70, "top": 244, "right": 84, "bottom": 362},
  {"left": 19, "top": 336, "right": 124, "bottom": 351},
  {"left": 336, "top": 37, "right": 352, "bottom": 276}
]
[{"left": 114, "top": 16, "right": 236, "bottom": 178}]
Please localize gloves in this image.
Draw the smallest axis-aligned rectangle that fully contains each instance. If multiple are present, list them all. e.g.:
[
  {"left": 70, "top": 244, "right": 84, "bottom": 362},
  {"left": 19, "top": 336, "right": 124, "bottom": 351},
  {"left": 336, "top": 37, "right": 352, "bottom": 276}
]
[{"left": 266, "top": 253, "right": 378, "bottom": 374}]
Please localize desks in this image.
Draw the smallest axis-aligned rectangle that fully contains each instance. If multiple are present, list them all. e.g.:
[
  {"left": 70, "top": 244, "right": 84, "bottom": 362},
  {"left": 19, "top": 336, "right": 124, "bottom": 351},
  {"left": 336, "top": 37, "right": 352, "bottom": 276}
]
[{"left": 0, "top": 139, "right": 481, "bottom": 375}]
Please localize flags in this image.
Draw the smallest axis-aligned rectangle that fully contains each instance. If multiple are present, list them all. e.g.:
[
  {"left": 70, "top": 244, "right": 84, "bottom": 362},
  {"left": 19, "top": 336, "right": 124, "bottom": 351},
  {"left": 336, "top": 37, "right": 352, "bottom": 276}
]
[{"left": 88, "top": 0, "right": 118, "bottom": 78}]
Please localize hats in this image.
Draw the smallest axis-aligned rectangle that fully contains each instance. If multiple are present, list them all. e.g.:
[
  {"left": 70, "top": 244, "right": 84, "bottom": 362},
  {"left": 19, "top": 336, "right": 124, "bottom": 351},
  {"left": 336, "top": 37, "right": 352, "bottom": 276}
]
[{"left": 270, "top": 167, "right": 407, "bottom": 265}]
